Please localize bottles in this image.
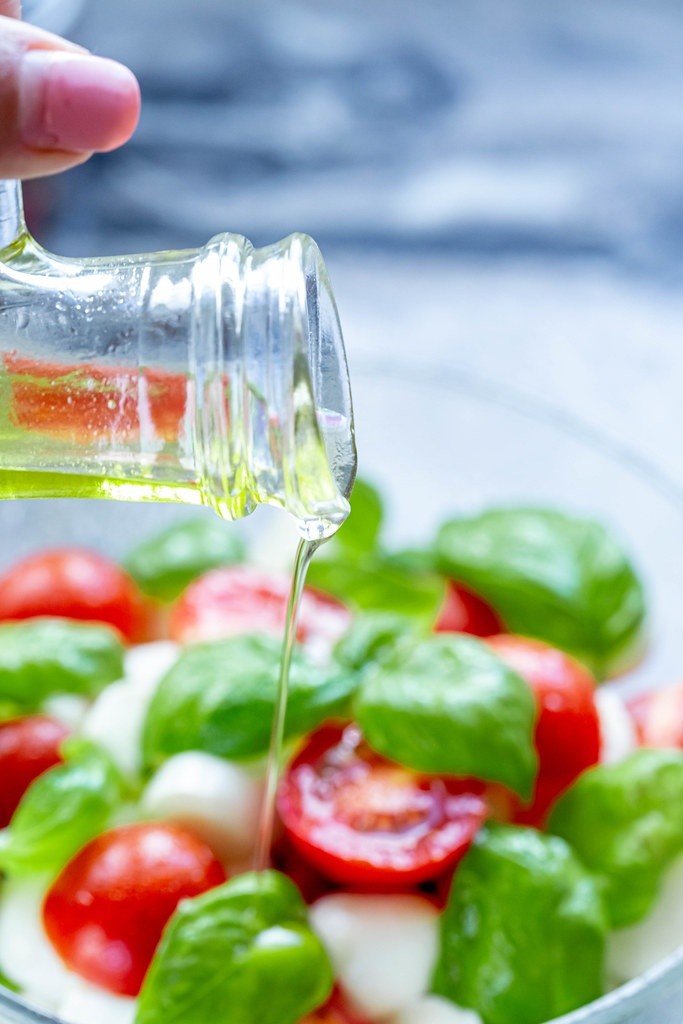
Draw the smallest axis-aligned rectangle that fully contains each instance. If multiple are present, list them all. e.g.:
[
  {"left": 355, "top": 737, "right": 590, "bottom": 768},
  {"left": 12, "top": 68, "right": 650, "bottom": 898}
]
[{"left": 1, "top": 177, "right": 360, "bottom": 522}]
